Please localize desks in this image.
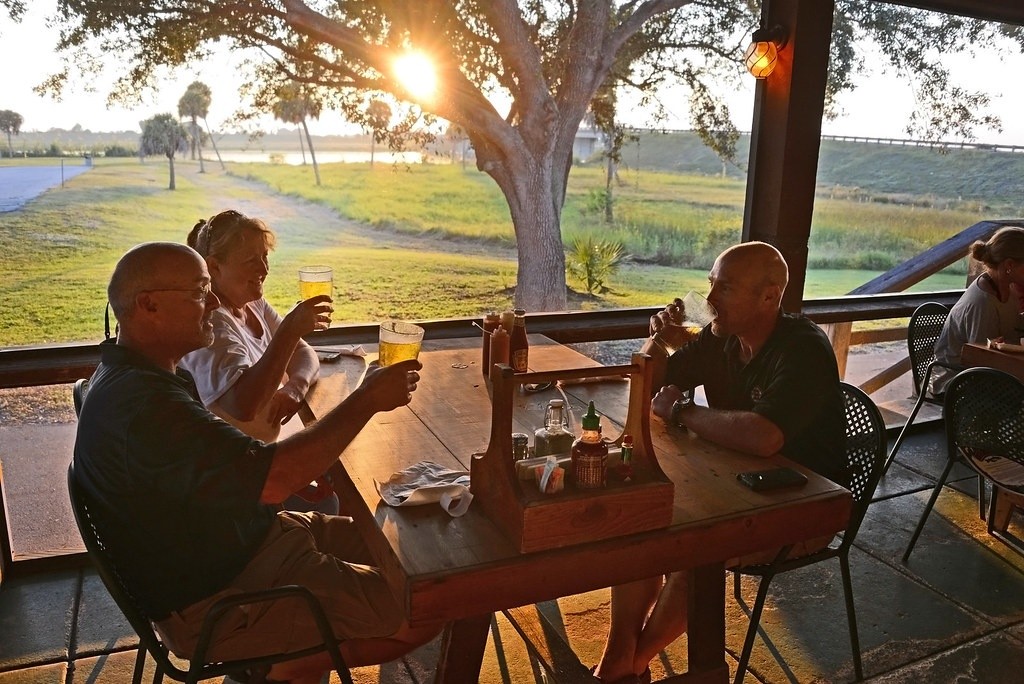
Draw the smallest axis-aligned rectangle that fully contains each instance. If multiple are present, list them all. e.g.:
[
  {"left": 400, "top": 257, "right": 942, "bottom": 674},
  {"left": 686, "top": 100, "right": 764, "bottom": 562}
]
[
  {"left": 960, "top": 341, "right": 1024, "bottom": 532},
  {"left": 283, "top": 333, "right": 852, "bottom": 684}
]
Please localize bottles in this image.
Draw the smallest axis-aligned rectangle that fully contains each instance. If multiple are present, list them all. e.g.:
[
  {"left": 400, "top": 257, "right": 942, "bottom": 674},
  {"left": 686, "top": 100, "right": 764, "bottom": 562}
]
[
  {"left": 534, "top": 399, "right": 573, "bottom": 457},
  {"left": 482, "top": 313, "right": 503, "bottom": 374},
  {"left": 571, "top": 400, "right": 608, "bottom": 492},
  {"left": 509, "top": 309, "right": 529, "bottom": 373},
  {"left": 500, "top": 311, "right": 513, "bottom": 336},
  {"left": 510, "top": 433, "right": 528, "bottom": 461},
  {"left": 489, "top": 325, "right": 509, "bottom": 379},
  {"left": 614, "top": 434, "right": 633, "bottom": 489}
]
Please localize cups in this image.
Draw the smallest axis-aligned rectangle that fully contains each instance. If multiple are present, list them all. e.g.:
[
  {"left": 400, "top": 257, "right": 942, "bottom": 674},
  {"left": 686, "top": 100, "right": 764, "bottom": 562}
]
[
  {"left": 651, "top": 290, "right": 718, "bottom": 357},
  {"left": 379, "top": 322, "right": 425, "bottom": 373},
  {"left": 298, "top": 265, "right": 333, "bottom": 331}
]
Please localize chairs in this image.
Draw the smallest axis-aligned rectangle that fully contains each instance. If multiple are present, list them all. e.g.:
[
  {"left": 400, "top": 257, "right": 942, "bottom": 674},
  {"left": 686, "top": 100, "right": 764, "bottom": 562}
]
[
  {"left": 882, "top": 302, "right": 985, "bottom": 519},
  {"left": 903, "top": 367, "right": 1023, "bottom": 561},
  {"left": 726, "top": 382, "right": 886, "bottom": 684},
  {"left": 68, "top": 378, "right": 354, "bottom": 684}
]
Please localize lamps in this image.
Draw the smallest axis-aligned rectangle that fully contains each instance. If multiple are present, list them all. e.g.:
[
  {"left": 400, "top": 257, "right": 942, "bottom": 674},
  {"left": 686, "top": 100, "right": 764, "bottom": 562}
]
[{"left": 745, "top": 17, "right": 789, "bottom": 79}]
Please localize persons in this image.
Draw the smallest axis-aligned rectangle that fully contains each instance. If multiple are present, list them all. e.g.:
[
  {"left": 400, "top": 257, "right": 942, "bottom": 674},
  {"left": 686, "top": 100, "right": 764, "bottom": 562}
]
[
  {"left": 177, "top": 210, "right": 339, "bottom": 515},
  {"left": 589, "top": 241, "right": 848, "bottom": 684},
  {"left": 73, "top": 242, "right": 444, "bottom": 684},
  {"left": 926, "top": 227, "right": 1024, "bottom": 515}
]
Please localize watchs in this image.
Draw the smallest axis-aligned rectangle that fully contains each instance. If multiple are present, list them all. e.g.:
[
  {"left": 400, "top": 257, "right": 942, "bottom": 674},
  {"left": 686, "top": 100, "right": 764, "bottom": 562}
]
[{"left": 671, "top": 397, "right": 696, "bottom": 429}]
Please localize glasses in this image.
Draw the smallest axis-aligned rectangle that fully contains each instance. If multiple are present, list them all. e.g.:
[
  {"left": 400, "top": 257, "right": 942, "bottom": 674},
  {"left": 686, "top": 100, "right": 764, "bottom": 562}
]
[{"left": 147, "top": 283, "right": 215, "bottom": 298}]
